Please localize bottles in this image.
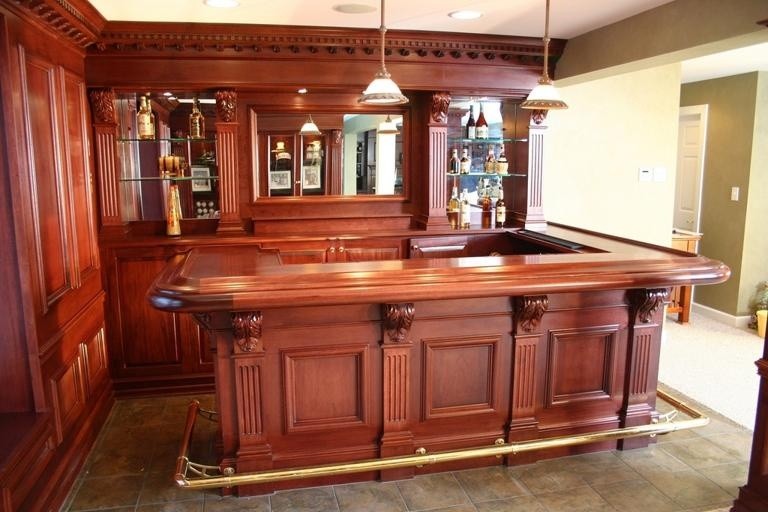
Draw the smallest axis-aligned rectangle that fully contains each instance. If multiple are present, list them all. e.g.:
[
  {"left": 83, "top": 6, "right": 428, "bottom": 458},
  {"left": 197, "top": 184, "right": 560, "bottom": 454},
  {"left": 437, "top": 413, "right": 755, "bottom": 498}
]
[
  {"left": 447, "top": 99, "right": 509, "bottom": 227},
  {"left": 122, "top": 90, "right": 217, "bottom": 238}
]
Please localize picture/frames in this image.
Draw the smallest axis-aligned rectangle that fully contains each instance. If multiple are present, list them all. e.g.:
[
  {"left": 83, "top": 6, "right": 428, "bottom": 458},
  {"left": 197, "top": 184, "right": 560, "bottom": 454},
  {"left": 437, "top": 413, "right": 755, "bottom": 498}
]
[{"left": 188, "top": 163, "right": 214, "bottom": 195}]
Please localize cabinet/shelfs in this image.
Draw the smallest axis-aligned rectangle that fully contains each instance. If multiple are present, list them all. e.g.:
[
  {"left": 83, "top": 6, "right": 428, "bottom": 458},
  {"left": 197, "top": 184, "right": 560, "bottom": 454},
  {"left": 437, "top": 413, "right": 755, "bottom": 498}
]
[
  {"left": 114, "top": 136, "right": 219, "bottom": 183},
  {"left": 406, "top": 235, "right": 466, "bottom": 258},
  {"left": 280, "top": 239, "right": 400, "bottom": 270},
  {"left": 445, "top": 136, "right": 527, "bottom": 180}
]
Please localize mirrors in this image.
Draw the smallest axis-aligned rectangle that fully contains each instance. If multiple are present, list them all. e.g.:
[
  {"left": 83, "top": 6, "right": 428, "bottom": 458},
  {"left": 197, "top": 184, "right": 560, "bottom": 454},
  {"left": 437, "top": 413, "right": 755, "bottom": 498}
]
[
  {"left": 444, "top": 94, "right": 518, "bottom": 214},
  {"left": 113, "top": 88, "right": 222, "bottom": 221},
  {"left": 244, "top": 103, "right": 413, "bottom": 207}
]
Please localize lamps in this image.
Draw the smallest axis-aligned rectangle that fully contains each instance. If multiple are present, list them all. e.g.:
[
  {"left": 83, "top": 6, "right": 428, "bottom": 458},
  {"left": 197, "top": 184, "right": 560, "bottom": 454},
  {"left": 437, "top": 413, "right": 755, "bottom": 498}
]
[
  {"left": 376, "top": 113, "right": 402, "bottom": 135},
  {"left": 519, "top": 1, "right": 571, "bottom": 112},
  {"left": 294, "top": 111, "right": 325, "bottom": 137},
  {"left": 354, "top": 2, "right": 410, "bottom": 108}
]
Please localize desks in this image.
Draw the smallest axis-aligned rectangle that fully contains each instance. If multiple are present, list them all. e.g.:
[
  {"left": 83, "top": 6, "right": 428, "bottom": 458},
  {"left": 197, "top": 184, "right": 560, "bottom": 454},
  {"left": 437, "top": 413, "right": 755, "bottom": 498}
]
[
  {"left": 665, "top": 226, "right": 704, "bottom": 326},
  {"left": 139, "top": 219, "right": 735, "bottom": 497}
]
